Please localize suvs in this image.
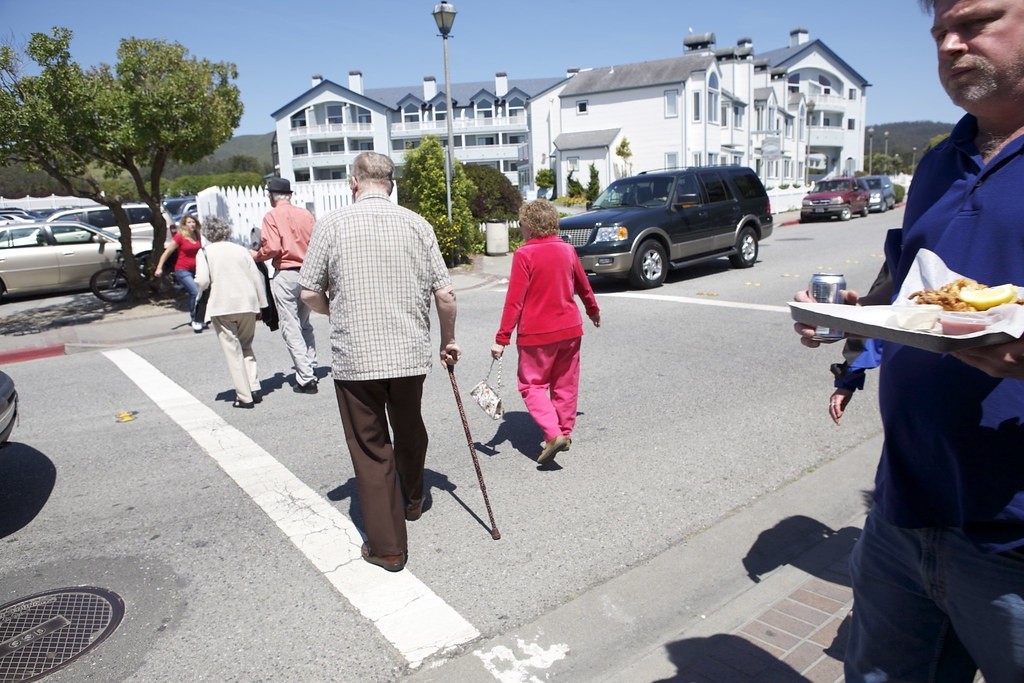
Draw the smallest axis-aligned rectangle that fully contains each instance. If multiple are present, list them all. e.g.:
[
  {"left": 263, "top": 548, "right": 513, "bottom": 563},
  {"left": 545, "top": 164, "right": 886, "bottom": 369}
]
[{"left": 557, "top": 163, "right": 774, "bottom": 291}]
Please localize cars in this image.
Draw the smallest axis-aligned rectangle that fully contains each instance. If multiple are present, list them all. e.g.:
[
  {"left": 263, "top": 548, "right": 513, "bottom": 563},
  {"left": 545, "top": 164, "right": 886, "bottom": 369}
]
[
  {"left": 0, "top": 220, "right": 172, "bottom": 306},
  {"left": 800, "top": 175, "right": 871, "bottom": 222},
  {"left": 861, "top": 174, "right": 897, "bottom": 213},
  {"left": 0, "top": 194, "right": 198, "bottom": 244}
]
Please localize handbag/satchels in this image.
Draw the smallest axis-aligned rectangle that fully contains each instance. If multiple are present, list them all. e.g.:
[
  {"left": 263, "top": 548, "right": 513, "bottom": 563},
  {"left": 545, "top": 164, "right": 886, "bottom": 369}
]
[{"left": 469, "top": 357, "right": 505, "bottom": 420}]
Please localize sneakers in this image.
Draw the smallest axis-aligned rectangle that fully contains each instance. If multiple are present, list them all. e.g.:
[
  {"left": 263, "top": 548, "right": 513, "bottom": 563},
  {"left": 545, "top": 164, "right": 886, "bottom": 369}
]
[{"left": 191, "top": 321, "right": 203, "bottom": 331}]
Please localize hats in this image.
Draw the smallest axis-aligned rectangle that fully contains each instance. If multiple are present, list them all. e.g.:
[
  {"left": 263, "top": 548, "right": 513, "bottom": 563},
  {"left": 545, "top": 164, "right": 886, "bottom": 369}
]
[{"left": 265, "top": 177, "right": 293, "bottom": 194}]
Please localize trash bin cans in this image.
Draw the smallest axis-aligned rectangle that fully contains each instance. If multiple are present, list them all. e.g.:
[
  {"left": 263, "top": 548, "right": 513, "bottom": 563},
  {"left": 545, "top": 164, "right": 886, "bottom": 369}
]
[{"left": 485, "top": 218, "right": 510, "bottom": 256}]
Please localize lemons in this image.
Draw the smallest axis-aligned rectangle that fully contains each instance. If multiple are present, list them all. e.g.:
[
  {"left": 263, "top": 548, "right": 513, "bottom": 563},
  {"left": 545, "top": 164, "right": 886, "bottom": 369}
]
[{"left": 958, "top": 283, "right": 1017, "bottom": 310}]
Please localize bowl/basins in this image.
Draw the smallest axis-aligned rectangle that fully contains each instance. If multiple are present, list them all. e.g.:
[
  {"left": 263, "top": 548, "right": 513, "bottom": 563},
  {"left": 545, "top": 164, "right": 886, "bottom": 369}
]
[
  {"left": 939, "top": 311, "right": 993, "bottom": 335},
  {"left": 892, "top": 304, "right": 943, "bottom": 329}
]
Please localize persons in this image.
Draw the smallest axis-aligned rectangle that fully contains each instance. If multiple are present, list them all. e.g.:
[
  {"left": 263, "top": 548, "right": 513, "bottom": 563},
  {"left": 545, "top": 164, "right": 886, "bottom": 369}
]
[
  {"left": 253, "top": 178, "right": 317, "bottom": 394},
  {"left": 828, "top": 259, "right": 892, "bottom": 425},
  {"left": 154, "top": 213, "right": 208, "bottom": 333},
  {"left": 194, "top": 214, "right": 269, "bottom": 409},
  {"left": 791, "top": 0, "right": 1024, "bottom": 683},
  {"left": 298, "top": 152, "right": 462, "bottom": 572},
  {"left": 492, "top": 200, "right": 602, "bottom": 466}
]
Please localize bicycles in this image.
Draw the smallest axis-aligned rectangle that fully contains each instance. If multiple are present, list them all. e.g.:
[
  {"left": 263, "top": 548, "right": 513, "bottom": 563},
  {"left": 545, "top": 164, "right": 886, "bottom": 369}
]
[{"left": 89, "top": 238, "right": 188, "bottom": 305}]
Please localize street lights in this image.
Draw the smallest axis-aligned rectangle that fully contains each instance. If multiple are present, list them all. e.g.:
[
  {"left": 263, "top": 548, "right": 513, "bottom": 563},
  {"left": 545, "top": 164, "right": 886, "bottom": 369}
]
[
  {"left": 867, "top": 127, "right": 875, "bottom": 176},
  {"left": 912, "top": 146, "right": 917, "bottom": 177},
  {"left": 431, "top": 0, "right": 459, "bottom": 270},
  {"left": 884, "top": 131, "right": 890, "bottom": 156}
]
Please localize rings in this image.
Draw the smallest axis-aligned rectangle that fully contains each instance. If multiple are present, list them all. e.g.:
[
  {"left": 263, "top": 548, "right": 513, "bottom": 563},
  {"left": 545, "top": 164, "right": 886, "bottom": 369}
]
[{"left": 829, "top": 403, "right": 834, "bottom": 406}]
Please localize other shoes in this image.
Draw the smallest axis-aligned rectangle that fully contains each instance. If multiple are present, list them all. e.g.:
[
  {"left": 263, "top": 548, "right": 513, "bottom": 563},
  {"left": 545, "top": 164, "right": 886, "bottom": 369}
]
[
  {"left": 559, "top": 437, "right": 572, "bottom": 452},
  {"left": 406, "top": 492, "right": 426, "bottom": 521},
  {"left": 293, "top": 380, "right": 318, "bottom": 394},
  {"left": 536, "top": 434, "right": 566, "bottom": 463},
  {"left": 232, "top": 400, "right": 254, "bottom": 409},
  {"left": 360, "top": 542, "right": 406, "bottom": 573},
  {"left": 251, "top": 390, "right": 262, "bottom": 404}
]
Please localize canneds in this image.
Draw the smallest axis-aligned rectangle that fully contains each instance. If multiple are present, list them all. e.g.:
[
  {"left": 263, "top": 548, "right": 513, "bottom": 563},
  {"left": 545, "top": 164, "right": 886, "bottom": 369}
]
[{"left": 809, "top": 272, "right": 846, "bottom": 342}]
